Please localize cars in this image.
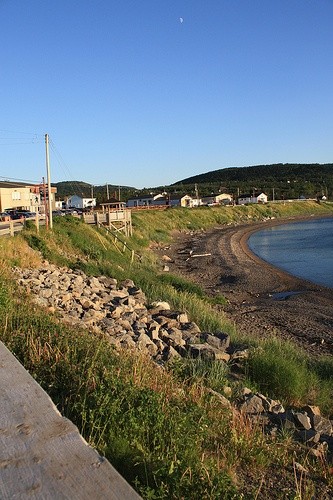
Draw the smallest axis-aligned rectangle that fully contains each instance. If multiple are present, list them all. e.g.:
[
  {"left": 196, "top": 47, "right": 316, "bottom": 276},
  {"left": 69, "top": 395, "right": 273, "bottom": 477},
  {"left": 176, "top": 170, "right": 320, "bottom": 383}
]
[
  {"left": 33, "top": 207, "right": 92, "bottom": 218},
  {"left": 13, "top": 212, "right": 33, "bottom": 219},
  {"left": 0, "top": 212, "right": 13, "bottom": 221}
]
